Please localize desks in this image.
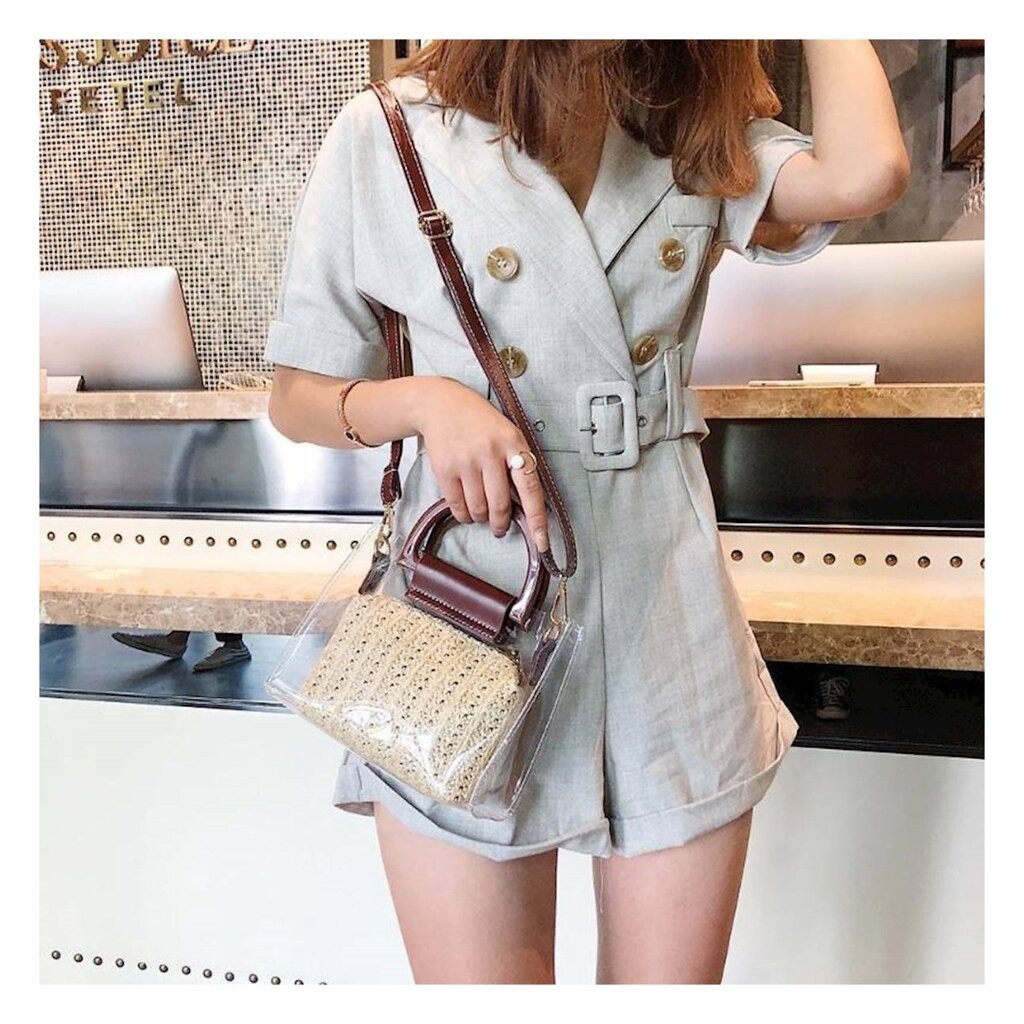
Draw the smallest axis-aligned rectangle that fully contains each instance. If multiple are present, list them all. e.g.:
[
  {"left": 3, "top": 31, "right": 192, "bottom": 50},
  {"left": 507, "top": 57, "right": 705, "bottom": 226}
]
[{"left": 41, "top": 384, "right": 985, "bottom": 672}]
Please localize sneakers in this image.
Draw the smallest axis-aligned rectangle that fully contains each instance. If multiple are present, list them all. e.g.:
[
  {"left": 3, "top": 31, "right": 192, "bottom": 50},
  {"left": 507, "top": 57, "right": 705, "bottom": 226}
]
[
  {"left": 189, "top": 647, "right": 251, "bottom": 673},
  {"left": 113, "top": 631, "right": 185, "bottom": 660}
]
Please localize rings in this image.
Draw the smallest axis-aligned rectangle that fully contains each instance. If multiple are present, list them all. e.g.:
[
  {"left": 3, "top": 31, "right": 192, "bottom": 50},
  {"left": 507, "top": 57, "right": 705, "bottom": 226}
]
[{"left": 507, "top": 449, "right": 537, "bottom": 475}]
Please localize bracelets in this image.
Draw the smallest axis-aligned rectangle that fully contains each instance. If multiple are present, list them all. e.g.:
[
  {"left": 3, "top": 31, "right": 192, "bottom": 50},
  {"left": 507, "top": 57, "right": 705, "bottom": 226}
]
[{"left": 337, "top": 378, "right": 385, "bottom": 449}]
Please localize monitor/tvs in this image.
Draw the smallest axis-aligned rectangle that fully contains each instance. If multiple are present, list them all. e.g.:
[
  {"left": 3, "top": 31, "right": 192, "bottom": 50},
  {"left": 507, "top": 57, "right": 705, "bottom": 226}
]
[{"left": 39, "top": 266, "right": 205, "bottom": 391}]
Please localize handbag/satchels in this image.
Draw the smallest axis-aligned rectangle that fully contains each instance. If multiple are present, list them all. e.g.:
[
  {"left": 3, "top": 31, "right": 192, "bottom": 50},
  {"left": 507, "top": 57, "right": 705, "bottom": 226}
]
[{"left": 268, "top": 498, "right": 586, "bottom": 820}]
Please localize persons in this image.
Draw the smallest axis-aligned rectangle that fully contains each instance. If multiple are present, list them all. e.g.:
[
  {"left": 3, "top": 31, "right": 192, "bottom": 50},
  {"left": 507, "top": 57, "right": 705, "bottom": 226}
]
[
  {"left": 111, "top": 630, "right": 251, "bottom": 671},
  {"left": 262, "top": 39, "right": 910, "bottom": 985}
]
